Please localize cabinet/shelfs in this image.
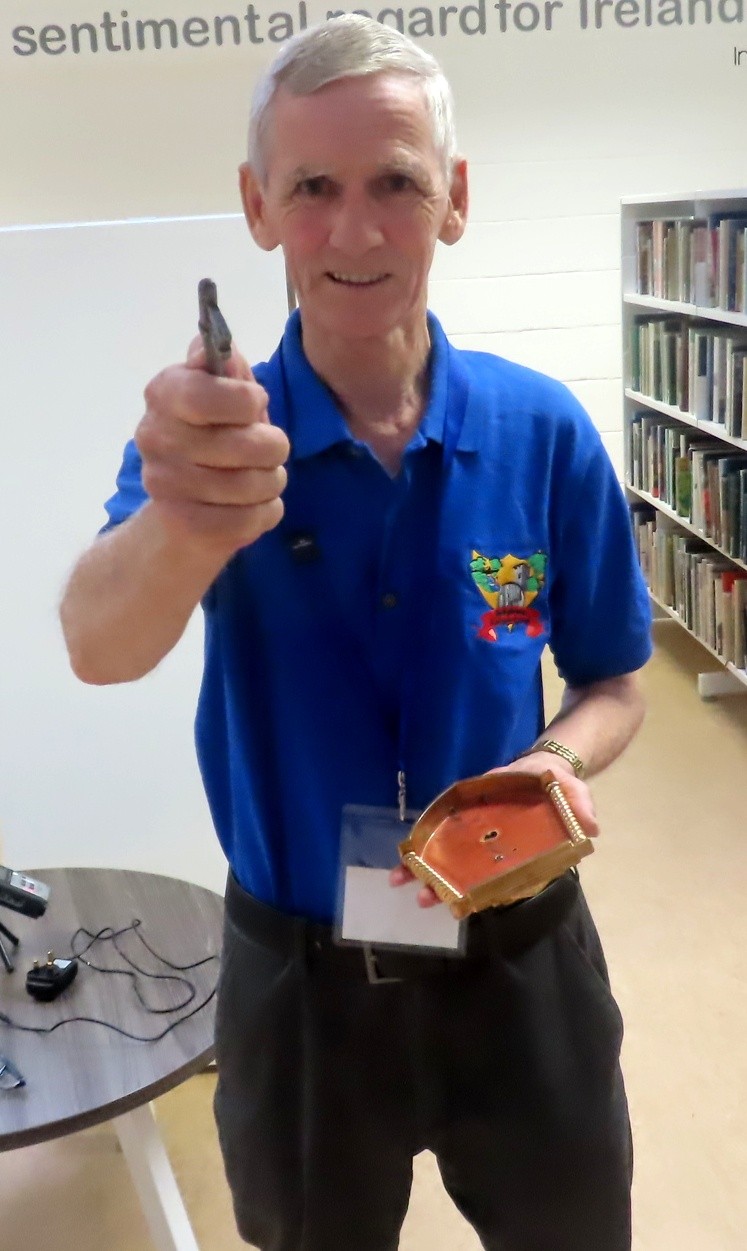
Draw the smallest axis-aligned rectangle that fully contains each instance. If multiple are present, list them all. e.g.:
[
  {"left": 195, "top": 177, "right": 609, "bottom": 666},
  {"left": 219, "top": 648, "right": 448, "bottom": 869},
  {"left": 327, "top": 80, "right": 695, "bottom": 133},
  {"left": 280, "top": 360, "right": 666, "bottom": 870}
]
[{"left": 619, "top": 192, "right": 747, "bottom": 701}]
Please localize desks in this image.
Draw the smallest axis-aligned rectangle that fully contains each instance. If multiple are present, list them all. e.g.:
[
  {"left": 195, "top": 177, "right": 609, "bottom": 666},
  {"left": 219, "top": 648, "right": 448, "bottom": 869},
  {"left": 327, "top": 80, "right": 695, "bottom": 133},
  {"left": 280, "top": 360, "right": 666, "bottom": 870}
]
[{"left": 0, "top": 867, "right": 227, "bottom": 1251}]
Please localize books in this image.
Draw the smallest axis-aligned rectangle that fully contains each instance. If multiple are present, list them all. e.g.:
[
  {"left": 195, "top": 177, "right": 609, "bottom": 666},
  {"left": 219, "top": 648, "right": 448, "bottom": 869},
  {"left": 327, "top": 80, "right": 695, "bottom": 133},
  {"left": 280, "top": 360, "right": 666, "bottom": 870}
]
[{"left": 631, "top": 217, "right": 747, "bottom": 672}]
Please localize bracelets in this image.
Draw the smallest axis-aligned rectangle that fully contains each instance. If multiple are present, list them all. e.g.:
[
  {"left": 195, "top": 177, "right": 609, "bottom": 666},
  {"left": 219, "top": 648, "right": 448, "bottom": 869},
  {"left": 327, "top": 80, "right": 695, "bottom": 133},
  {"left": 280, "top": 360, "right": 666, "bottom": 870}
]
[{"left": 526, "top": 739, "right": 585, "bottom": 780}]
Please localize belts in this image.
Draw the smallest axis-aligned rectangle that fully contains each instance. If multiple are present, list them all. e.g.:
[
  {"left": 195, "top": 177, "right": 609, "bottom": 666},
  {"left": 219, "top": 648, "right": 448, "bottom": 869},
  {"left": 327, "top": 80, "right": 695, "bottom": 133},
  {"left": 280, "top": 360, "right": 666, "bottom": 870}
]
[{"left": 222, "top": 864, "right": 579, "bottom": 988}]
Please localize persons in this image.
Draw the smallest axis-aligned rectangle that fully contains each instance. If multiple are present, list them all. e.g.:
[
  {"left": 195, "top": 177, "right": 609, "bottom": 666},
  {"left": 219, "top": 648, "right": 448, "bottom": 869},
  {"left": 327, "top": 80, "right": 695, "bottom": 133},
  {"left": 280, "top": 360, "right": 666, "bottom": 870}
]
[{"left": 61, "top": 14, "right": 653, "bottom": 1251}]
